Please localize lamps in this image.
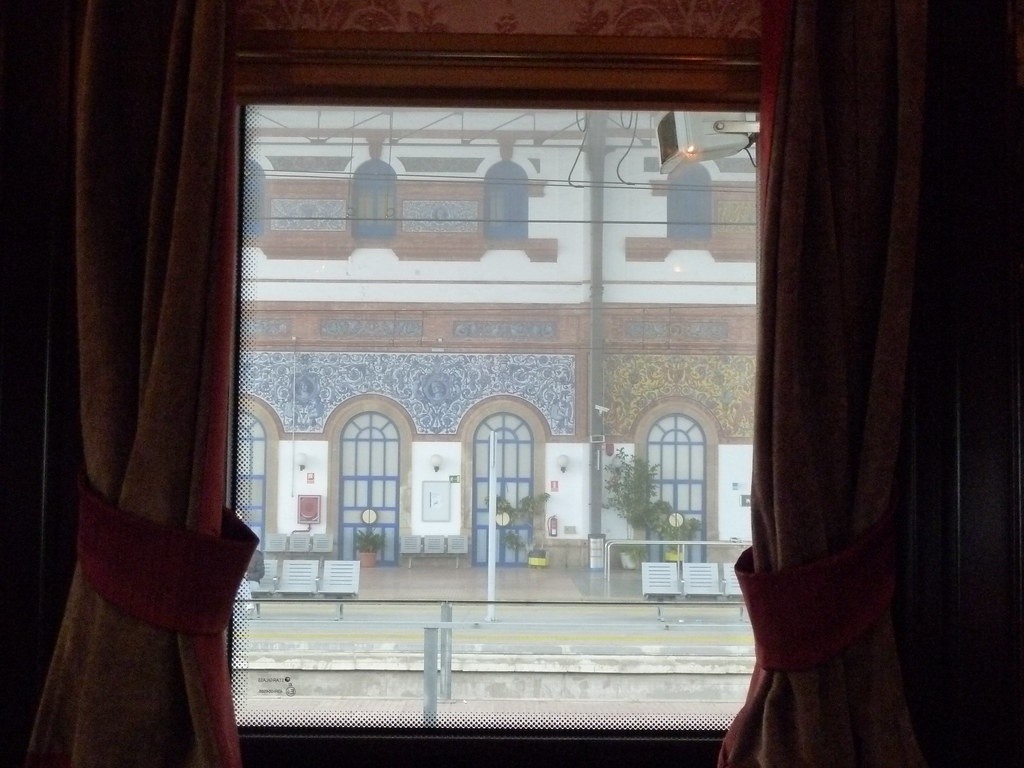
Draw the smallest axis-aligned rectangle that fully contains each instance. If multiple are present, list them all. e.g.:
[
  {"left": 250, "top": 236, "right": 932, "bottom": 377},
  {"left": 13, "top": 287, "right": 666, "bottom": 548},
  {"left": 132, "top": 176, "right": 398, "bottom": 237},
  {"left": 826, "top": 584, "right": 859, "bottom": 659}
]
[{"left": 658, "top": 112, "right": 760, "bottom": 175}]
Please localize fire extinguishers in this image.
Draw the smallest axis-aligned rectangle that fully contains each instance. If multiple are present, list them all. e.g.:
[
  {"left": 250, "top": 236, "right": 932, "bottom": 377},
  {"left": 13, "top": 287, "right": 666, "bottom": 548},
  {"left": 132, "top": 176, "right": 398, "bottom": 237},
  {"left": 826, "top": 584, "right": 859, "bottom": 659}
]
[{"left": 547, "top": 515, "right": 557, "bottom": 536}]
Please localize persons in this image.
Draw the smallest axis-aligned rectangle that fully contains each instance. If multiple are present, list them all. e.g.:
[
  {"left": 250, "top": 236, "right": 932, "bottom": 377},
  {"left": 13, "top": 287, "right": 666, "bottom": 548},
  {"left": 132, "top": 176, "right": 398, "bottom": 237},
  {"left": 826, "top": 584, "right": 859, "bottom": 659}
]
[{"left": 244, "top": 550, "right": 265, "bottom": 614}]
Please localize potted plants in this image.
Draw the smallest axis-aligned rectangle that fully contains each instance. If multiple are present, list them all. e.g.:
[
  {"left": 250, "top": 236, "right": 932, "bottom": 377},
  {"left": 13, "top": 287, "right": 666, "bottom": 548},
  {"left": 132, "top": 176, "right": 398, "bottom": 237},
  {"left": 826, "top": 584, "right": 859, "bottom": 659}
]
[
  {"left": 602, "top": 447, "right": 703, "bottom": 569},
  {"left": 485, "top": 493, "right": 551, "bottom": 558},
  {"left": 354, "top": 528, "right": 385, "bottom": 567}
]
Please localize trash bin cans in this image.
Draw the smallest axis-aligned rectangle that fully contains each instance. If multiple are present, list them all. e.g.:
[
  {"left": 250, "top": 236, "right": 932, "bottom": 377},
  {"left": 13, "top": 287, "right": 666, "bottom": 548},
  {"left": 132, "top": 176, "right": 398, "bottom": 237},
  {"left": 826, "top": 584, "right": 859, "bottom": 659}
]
[{"left": 587, "top": 534, "right": 606, "bottom": 569}]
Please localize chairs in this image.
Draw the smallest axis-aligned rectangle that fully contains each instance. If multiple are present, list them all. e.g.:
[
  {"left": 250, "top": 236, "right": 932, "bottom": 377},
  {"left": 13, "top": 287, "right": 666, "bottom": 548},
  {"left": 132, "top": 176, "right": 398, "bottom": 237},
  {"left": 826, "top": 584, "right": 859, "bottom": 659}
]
[
  {"left": 447, "top": 535, "right": 468, "bottom": 569},
  {"left": 721, "top": 562, "right": 751, "bottom": 623},
  {"left": 641, "top": 562, "right": 680, "bottom": 621},
  {"left": 311, "top": 533, "right": 333, "bottom": 567},
  {"left": 249, "top": 561, "right": 278, "bottom": 620},
  {"left": 423, "top": 535, "right": 445, "bottom": 554},
  {"left": 315, "top": 560, "right": 361, "bottom": 621},
  {"left": 679, "top": 563, "right": 726, "bottom": 599},
  {"left": 287, "top": 534, "right": 311, "bottom": 553},
  {"left": 263, "top": 534, "right": 288, "bottom": 560},
  {"left": 273, "top": 560, "right": 321, "bottom": 598},
  {"left": 400, "top": 535, "right": 421, "bottom": 568}
]
